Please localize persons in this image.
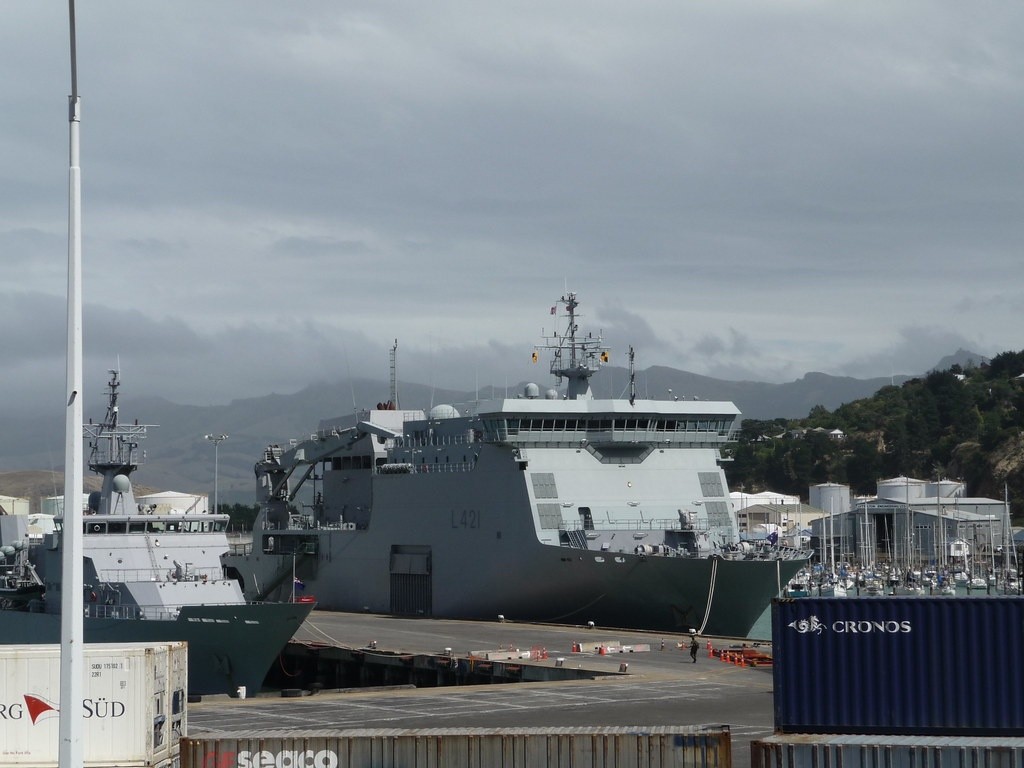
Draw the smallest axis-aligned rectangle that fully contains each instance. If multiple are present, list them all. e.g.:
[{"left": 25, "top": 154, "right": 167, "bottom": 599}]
[{"left": 688, "top": 636, "right": 700, "bottom": 664}]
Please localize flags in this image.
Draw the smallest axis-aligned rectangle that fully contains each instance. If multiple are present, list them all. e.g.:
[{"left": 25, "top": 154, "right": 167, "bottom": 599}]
[
  {"left": 765, "top": 528, "right": 778, "bottom": 548},
  {"left": 530, "top": 350, "right": 540, "bottom": 365},
  {"left": 600, "top": 350, "right": 610, "bottom": 365},
  {"left": 549, "top": 306, "right": 556, "bottom": 315},
  {"left": 295, "top": 577, "right": 306, "bottom": 591}
]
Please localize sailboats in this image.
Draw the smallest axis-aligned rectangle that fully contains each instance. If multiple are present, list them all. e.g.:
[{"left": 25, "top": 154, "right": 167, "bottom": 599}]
[{"left": 732, "top": 473, "right": 1022, "bottom": 597}]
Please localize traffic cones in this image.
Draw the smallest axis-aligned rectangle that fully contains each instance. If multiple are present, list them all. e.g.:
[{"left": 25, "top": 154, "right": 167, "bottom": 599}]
[
  {"left": 681, "top": 641, "right": 685, "bottom": 650},
  {"left": 726, "top": 650, "right": 731, "bottom": 663},
  {"left": 719, "top": 647, "right": 725, "bottom": 661},
  {"left": 740, "top": 654, "right": 746, "bottom": 667},
  {"left": 660, "top": 638, "right": 665, "bottom": 651},
  {"left": 707, "top": 637, "right": 711, "bottom": 649},
  {"left": 734, "top": 651, "right": 738, "bottom": 665},
  {"left": 572, "top": 640, "right": 576, "bottom": 652},
  {"left": 499, "top": 642, "right": 548, "bottom": 661},
  {"left": 600, "top": 641, "right": 605, "bottom": 655},
  {"left": 708, "top": 646, "right": 714, "bottom": 658}
]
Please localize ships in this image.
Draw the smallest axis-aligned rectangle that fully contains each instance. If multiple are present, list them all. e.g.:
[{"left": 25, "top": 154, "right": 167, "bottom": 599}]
[
  {"left": 0, "top": 356, "right": 318, "bottom": 698},
  {"left": 218, "top": 284, "right": 816, "bottom": 638}
]
